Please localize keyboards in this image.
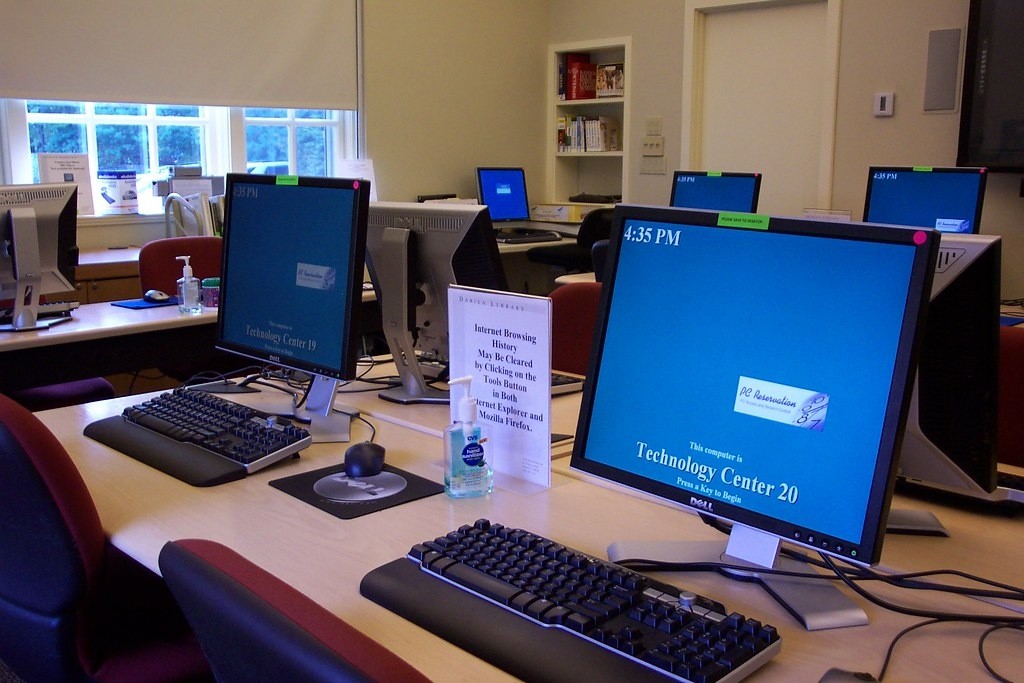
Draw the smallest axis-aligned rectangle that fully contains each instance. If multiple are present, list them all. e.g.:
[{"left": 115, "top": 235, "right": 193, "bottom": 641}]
[
  {"left": 891, "top": 468, "right": 1024, "bottom": 507},
  {"left": 82, "top": 389, "right": 313, "bottom": 487},
  {"left": 496, "top": 235, "right": 559, "bottom": 245},
  {"left": 417, "top": 352, "right": 585, "bottom": 395},
  {"left": 359, "top": 518, "right": 782, "bottom": 683},
  {"left": 0, "top": 301, "right": 80, "bottom": 323}
]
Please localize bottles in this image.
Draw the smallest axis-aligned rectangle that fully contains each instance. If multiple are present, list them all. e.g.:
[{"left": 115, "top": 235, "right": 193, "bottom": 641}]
[{"left": 202, "top": 278, "right": 220, "bottom": 307}]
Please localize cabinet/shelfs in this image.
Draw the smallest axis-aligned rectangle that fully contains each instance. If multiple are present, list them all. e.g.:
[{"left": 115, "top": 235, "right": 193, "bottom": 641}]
[
  {"left": 0, "top": 243, "right": 177, "bottom": 397},
  {"left": 547, "top": 34, "right": 632, "bottom": 203}
]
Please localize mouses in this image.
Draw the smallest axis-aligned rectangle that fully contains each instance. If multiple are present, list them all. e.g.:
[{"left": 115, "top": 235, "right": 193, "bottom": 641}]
[
  {"left": 545, "top": 230, "right": 563, "bottom": 240},
  {"left": 819, "top": 667, "right": 881, "bottom": 683},
  {"left": 144, "top": 290, "right": 169, "bottom": 303},
  {"left": 344, "top": 440, "right": 386, "bottom": 477}
]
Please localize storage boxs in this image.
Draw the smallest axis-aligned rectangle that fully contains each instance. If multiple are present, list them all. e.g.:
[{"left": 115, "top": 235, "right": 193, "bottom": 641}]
[
  {"left": 97, "top": 169, "right": 138, "bottom": 215},
  {"left": 530, "top": 202, "right": 615, "bottom": 224}
]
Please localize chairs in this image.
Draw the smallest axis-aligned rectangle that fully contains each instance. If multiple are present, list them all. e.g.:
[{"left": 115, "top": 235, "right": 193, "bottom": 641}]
[
  {"left": 0, "top": 295, "right": 116, "bottom": 411},
  {"left": 998, "top": 325, "right": 1024, "bottom": 468},
  {"left": 548, "top": 282, "right": 602, "bottom": 375},
  {"left": 0, "top": 393, "right": 214, "bottom": 683},
  {"left": 159, "top": 538, "right": 433, "bottom": 683},
  {"left": 138, "top": 235, "right": 222, "bottom": 298},
  {"left": 526, "top": 209, "right": 613, "bottom": 274}
]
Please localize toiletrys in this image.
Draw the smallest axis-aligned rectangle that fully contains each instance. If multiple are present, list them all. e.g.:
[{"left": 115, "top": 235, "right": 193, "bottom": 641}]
[
  {"left": 442, "top": 376, "right": 493, "bottom": 500},
  {"left": 175, "top": 255, "right": 202, "bottom": 314}
]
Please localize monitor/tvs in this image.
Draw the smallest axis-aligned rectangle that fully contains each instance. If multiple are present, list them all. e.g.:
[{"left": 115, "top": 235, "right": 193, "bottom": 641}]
[
  {"left": 214, "top": 174, "right": 371, "bottom": 441},
  {"left": 365, "top": 199, "right": 512, "bottom": 405},
  {"left": 475, "top": 166, "right": 531, "bottom": 237},
  {"left": 567, "top": 165, "right": 1002, "bottom": 631},
  {"left": 0, "top": 183, "right": 80, "bottom": 332}
]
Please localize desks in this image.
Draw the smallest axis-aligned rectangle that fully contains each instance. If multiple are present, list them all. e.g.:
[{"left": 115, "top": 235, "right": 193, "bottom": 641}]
[
  {"left": 33, "top": 377, "right": 1024, "bottom": 683},
  {"left": 247, "top": 349, "right": 1024, "bottom": 615},
  {"left": 498, "top": 236, "right": 578, "bottom": 254},
  {"left": 0, "top": 278, "right": 378, "bottom": 394}
]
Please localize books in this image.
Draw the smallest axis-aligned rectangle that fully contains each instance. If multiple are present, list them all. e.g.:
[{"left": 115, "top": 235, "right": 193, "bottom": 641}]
[
  {"left": 558, "top": 52, "right": 597, "bottom": 100},
  {"left": 181, "top": 192, "right": 226, "bottom": 238},
  {"left": 558, "top": 113, "right": 621, "bottom": 152}
]
